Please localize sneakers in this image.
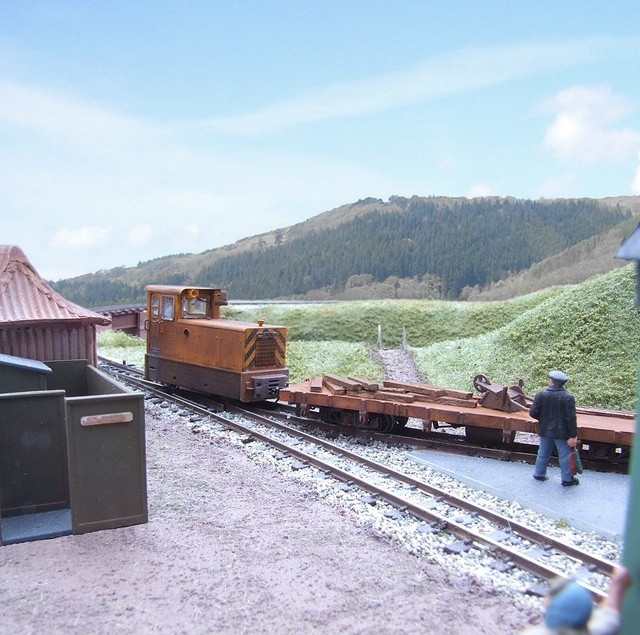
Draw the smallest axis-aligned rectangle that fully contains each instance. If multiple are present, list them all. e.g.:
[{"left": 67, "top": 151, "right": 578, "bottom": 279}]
[
  {"left": 533, "top": 474, "right": 548, "bottom": 480},
  {"left": 562, "top": 478, "right": 578, "bottom": 486}
]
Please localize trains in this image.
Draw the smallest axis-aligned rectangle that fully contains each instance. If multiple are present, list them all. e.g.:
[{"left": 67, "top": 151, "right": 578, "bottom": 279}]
[{"left": 143, "top": 284, "right": 634, "bottom": 460}]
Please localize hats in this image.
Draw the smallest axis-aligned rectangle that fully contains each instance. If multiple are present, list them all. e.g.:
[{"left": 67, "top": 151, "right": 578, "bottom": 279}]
[{"left": 548, "top": 371, "right": 568, "bottom": 382}]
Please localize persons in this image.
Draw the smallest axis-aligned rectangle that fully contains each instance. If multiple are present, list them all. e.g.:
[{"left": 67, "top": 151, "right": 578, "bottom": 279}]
[
  {"left": 529, "top": 370, "right": 579, "bottom": 486},
  {"left": 521, "top": 564, "right": 633, "bottom": 635}
]
